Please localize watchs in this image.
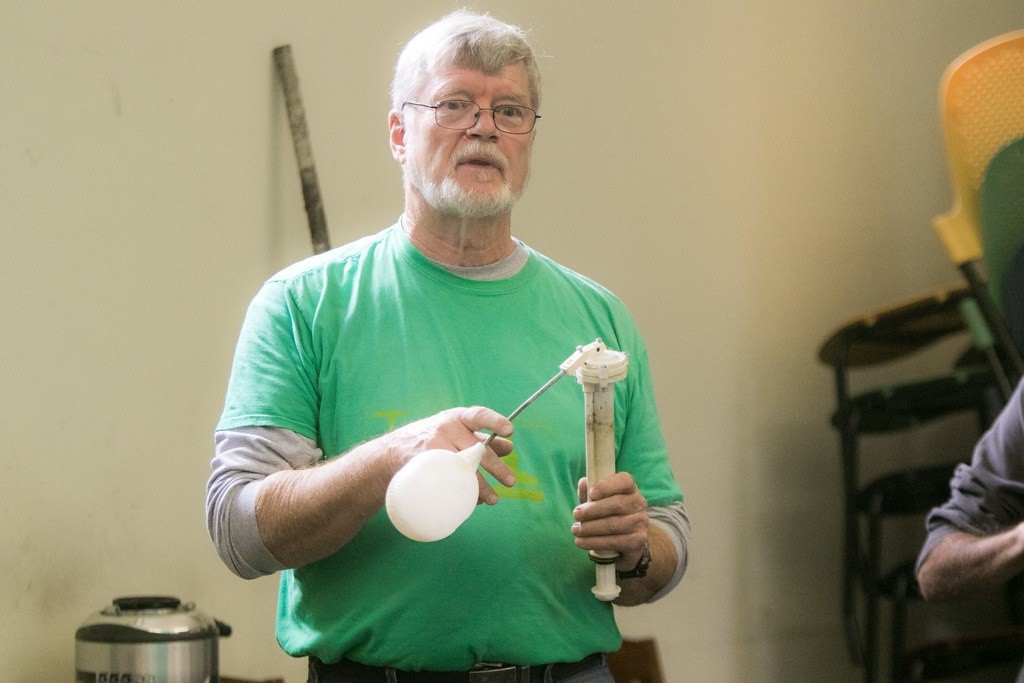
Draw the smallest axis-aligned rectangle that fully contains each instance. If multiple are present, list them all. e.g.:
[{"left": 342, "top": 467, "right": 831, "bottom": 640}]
[{"left": 615, "top": 547, "right": 652, "bottom": 580}]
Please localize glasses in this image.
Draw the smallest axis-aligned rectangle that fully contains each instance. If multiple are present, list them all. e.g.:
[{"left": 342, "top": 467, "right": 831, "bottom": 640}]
[{"left": 401, "top": 99, "right": 542, "bottom": 135}]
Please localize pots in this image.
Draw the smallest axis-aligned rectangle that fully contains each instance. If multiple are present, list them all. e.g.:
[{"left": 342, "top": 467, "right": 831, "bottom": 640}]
[{"left": 75, "top": 597, "right": 232, "bottom": 683}]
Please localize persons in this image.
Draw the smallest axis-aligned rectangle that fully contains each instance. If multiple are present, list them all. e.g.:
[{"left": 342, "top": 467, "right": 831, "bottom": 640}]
[
  {"left": 912, "top": 374, "right": 1024, "bottom": 607},
  {"left": 202, "top": 9, "right": 695, "bottom": 683}
]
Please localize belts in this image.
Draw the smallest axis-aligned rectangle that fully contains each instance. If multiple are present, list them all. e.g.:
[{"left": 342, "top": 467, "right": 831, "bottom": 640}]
[{"left": 309, "top": 653, "right": 602, "bottom": 683}]
